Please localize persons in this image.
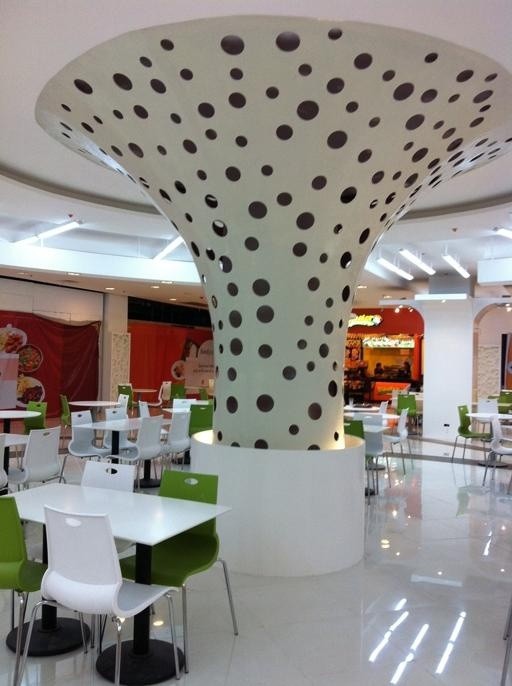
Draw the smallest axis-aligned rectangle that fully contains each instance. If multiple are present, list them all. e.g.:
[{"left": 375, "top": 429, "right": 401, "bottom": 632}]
[
  {"left": 372, "top": 361, "right": 384, "bottom": 375},
  {"left": 403, "top": 360, "right": 410, "bottom": 373}
]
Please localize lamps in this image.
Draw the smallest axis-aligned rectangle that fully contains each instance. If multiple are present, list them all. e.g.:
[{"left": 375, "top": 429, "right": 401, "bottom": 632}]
[
  {"left": 374, "top": 227, "right": 512, "bottom": 281},
  {"left": 12, "top": 221, "right": 82, "bottom": 245},
  {"left": 154, "top": 236, "right": 183, "bottom": 262}
]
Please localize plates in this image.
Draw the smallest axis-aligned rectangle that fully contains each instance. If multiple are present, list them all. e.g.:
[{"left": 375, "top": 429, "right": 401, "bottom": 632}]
[
  {"left": 354, "top": 404, "right": 372, "bottom": 408},
  {"left": 171, "top": 360, "right": 186, "bottom": 381},
  {"left": 1, "top": 325, "right": 47, "bottom": 408}
]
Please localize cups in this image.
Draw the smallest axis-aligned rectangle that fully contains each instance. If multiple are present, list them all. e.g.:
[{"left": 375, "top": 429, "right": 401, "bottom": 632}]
[
  {"left": 209, "top": 379, "right": 214, "bottom": 394},
  {"left": 349, "top": 397, "right": 353, "bottom": 407}
]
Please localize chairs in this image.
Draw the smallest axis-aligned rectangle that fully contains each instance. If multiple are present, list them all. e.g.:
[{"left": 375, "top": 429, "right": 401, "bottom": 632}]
[{"left": 344, "top": 391, "right": 512, "bottom": 504}]
